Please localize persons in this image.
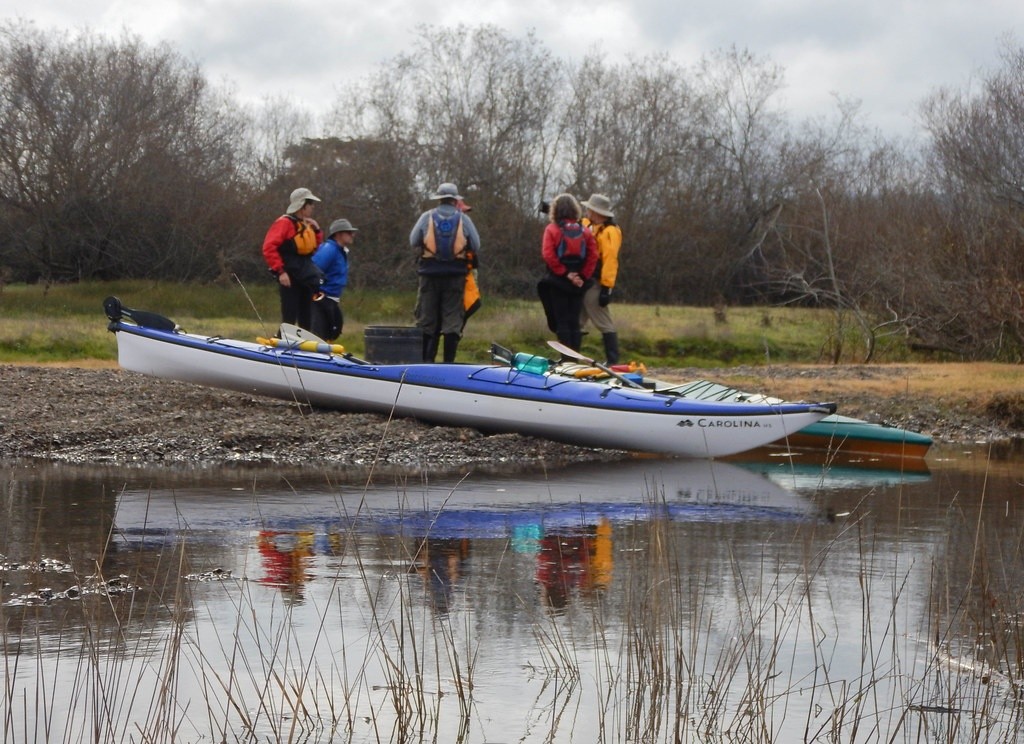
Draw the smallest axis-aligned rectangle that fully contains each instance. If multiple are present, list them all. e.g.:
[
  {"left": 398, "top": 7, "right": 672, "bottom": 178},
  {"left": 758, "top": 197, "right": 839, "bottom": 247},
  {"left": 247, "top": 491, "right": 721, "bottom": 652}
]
[
  {"left": 408, "top": 182, "right": 481, "bottom": 365},
  {"left": 538, "top": 193, "right": 623, "bottom": 368},
  {"left": 310, "top": 219, "right": 359, "bottom": 340},
  {"left": 262, "top": 187, "right": 324, "bottom": 340},
  {"left": 542, "top": 192, "right": 600, "bottom": 366}
]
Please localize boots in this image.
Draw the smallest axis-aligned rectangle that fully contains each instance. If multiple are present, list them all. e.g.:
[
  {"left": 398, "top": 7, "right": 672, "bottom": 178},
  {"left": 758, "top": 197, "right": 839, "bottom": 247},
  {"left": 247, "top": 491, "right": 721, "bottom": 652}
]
[
  {"left": 422, "top": 333, "right": 458, "bottom": 363},
  {"left": 555, "top": 333, "right": 582, "bottom": 362},
  {"left": 602, "top": 332, "right": 621, "bottom": 366}
]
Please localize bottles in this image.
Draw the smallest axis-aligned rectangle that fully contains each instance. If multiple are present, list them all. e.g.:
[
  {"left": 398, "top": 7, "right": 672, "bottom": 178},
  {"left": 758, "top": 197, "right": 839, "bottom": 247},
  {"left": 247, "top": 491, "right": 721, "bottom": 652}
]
[
  {"left": 511, "top": 352, "right": 549, "bottom": 374},
  {"left": 465, "top": 245, "right": 473, "bottom": 269}
]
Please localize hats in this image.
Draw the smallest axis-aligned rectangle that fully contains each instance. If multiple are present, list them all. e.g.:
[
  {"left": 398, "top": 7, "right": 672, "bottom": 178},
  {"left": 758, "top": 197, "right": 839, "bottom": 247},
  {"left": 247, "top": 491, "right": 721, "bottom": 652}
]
[
  {"left": 328, "top": 219, "right": 358, "bottom": 236},
  {"left": 428, "top": 183, "right": 464, "bottom": 200},
  {"left": 457, "top": 201, "right": 471, "bottom": 212},
  {"left": 580, "top": 193, "right": 614, "bottom": 217},
  {"left": 286, "top": 188, "right": 321, "bottom": 214}
]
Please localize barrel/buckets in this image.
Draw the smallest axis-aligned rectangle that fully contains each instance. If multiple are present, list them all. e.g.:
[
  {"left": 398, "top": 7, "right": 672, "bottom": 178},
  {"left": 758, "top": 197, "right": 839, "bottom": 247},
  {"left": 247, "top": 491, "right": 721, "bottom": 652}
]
[{"left": 364, "top": 324, "right": 426, "bottom": 365}]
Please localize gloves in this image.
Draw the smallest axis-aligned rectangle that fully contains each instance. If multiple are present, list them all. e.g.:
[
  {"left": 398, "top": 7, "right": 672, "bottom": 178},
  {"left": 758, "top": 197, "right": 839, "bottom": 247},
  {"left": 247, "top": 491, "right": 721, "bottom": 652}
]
[
  {"left": 600, "top": 286, "right": 612, "bottom": 307},
  {"left": 538, "top": 200, "right": 550, "bottom": 213}
]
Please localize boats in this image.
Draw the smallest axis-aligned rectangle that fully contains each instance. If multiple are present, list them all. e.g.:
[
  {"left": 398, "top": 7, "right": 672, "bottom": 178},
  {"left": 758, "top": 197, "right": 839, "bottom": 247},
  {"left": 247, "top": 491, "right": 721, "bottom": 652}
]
[
  {"left": 100, "top": 294, "right": 837, "bottom": 462},
  {"left": 112, "top": 459, "right": 838, "bottom": 542},
  {"left": 768, "top": 412, "right": 933, "bottom": 460}
]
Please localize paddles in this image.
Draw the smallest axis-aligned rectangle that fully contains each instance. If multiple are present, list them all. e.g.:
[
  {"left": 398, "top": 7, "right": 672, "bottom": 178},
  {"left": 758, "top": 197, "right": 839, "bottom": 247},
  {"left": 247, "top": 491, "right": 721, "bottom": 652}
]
[
  {"left": 547, "top": 340, "right": 646, "bottom": 392},
  {"left": 280, "top": 322, "right": 372, "bottom": 366}
]
